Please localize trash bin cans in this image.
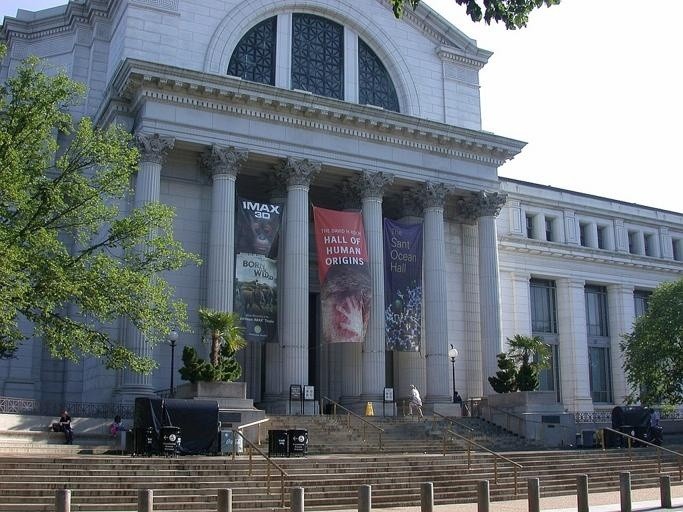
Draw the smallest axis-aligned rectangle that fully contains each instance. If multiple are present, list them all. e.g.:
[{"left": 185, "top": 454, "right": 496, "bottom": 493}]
[
  {"left": 159, "top": 426, "right": 182, "bottom": 458},
  {"left": 221, "top": 430, "right": 244, "bottom": 456},
  {"left": 582, "top": 430, "right": 595, "bottom": 448},
  {"left": 268, "top": 430, "right": 308, "bottom": 457},
  {"left": 131, "top": 425, "right": 153, "bottom": 458},
  {"left": 602, "top": 425, "right": 651, "bottom": 448}
]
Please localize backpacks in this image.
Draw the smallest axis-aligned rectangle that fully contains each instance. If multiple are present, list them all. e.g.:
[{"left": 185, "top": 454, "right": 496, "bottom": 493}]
[
  {"left": 111, "top": 422, "right": 118, "bottom": 438},
  {"left": 53, "top": 423, "right": 63, "bottom": 432}
]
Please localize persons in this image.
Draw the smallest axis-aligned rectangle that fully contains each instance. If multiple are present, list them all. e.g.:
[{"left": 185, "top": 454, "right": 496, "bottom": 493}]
[
  {"left": 111, "top": 415, "right": 122, "bottom": 440},
  {"left": 408, "top": 385, "right": 423, "bottom": 418},
  {"left": 455, "top": 391, "right": 462, "bottom": 401},
  {"left": 634, "top": 407, "right": 663, "bottom": 448},
  {"left": 59, "top": 409, "right": 73, "bottom": 445}
]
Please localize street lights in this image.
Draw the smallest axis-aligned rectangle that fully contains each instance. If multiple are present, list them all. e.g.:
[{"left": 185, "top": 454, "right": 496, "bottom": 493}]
[
  {"left": 167, "top": 327, "right": 177, "bottom": 397},
  {"left": 447, "top": 344, "right": 459, "bottom": 402}
]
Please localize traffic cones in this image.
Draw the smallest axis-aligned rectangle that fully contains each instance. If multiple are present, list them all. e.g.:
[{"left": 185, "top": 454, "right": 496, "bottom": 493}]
[{"left": 364, "top": 401, "right": 374, "bottom": 416}]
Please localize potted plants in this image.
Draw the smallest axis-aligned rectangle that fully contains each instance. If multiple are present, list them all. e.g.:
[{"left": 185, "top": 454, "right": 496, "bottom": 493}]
[
  {"left": 488, "top": 334, "right": 558, "bottom": 407},
  {"left": 175, "top": 306, "right": 247, "bottom": 398}
]
